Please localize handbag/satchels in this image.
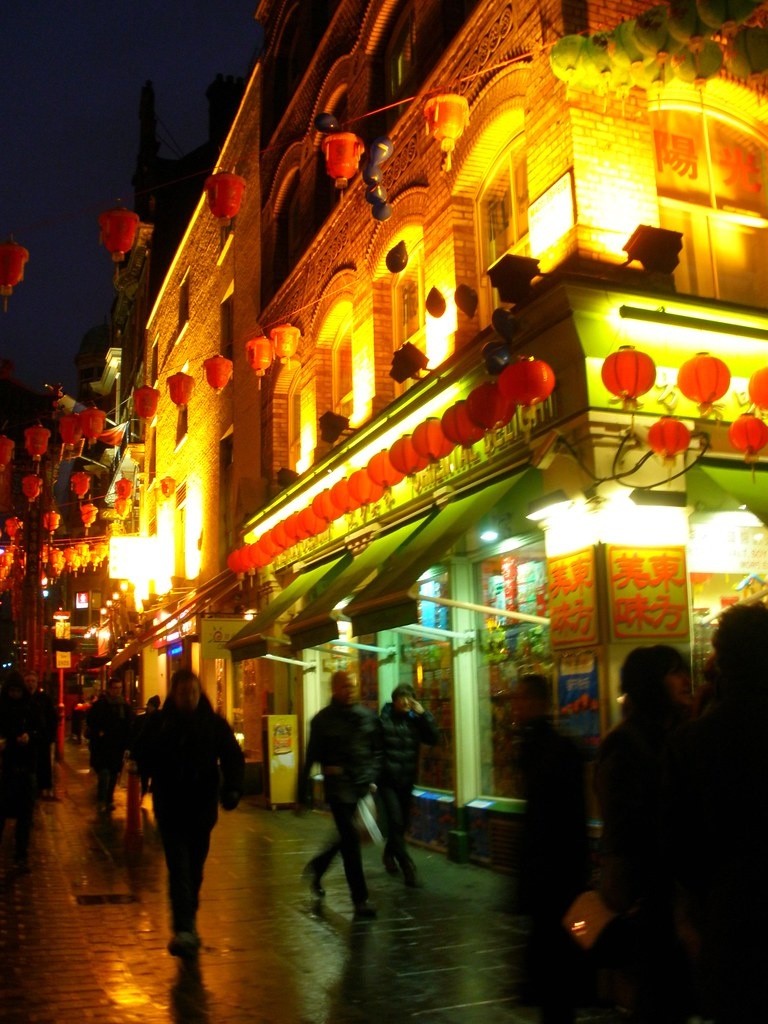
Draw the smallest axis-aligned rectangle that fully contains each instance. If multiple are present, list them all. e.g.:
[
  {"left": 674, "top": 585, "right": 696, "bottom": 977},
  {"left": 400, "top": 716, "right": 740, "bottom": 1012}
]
[{"left": 558, "top": 890, "right": 616, "bottom": 954}]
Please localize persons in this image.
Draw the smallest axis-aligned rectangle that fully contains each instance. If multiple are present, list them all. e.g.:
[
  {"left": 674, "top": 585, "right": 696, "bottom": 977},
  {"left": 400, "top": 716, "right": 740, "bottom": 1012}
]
[
  {"left": 563, "top": 603, "right": 768, "bottom": 1024},
  {"left": 72, "top": 678, "right": 162, "bottom": 812},
  {"left": 510, "top": 674, "right": 587, "bottom": 1024},
  {"left": 147, "top": 668, "right": 247, "bottom": 956},
  {"left": 295, "top": 670, "right": 381, "bottom": 920},
  {"left": 372, "top": 683, "right": 439, "bottom": 888},
  {"left": 0, "top": 670, "right": 62, "bottom": 871}
]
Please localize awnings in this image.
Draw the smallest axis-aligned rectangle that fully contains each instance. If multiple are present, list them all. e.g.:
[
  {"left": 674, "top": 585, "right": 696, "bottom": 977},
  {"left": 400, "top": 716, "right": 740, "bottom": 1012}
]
[{"left": 224, "top": 464, "right": 533, "bottom": 664}]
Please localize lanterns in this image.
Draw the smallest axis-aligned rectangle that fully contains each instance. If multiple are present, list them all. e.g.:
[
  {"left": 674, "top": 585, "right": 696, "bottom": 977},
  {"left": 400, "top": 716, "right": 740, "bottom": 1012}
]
[
  {"left": 228, "top": 352, "right": 557, "bottom": 576},
  {"left": 0, "top": 93, "right": 476, "bottom": 314},
  {"left": 0, "top": 323, "right": 304, "bottom": 583},
  {"left": 602, "top": 343, "right": 768, "bottom": 488},
  {"left": 549, "top": 0, "right": 768, "bottom": 118}
]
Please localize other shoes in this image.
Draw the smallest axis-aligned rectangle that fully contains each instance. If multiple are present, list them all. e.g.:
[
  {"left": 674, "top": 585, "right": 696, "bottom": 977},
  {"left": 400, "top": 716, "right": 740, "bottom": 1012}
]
[
  {"left": 351, "top": 900, "right": 382, "bottom": 914},
  {"left": 382, "top": 852, "right": 402, "bottom": 875},
  {"left": 403, "top": 869, "right": 426, "bottom": 888},
  {"left": 304, "top": 864, "right": 327, "bottom": 897},
  {"left": 167, "top": 933, "right": 203, "bottom": 960}
]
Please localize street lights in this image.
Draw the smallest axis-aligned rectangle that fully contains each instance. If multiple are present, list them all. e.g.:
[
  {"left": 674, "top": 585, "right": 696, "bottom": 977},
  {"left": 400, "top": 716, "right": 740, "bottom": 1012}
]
[{"left": 53, "top": 610, "right": 70, "bottom": 758}]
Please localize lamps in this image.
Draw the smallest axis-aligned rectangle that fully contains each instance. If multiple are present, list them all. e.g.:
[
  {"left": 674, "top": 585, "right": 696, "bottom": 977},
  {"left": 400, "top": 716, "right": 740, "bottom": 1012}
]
[
  {"left": 388, "top": 342, "right": 436, "bottom": 384},
  {"left": 485, "top": 254, "right": 550, "bottom": 305},
  {"left": 523, "top": 488, "right": 569, "bottom": 522},
  {"left": 317, "top": 411, "right": 361, "bottom": 444},
  {"left": 277, "top": 468, "right": 300, "bottom": 488},
  {"left": 618, "top": 224, "right": 683, "bottom": 275}
]
